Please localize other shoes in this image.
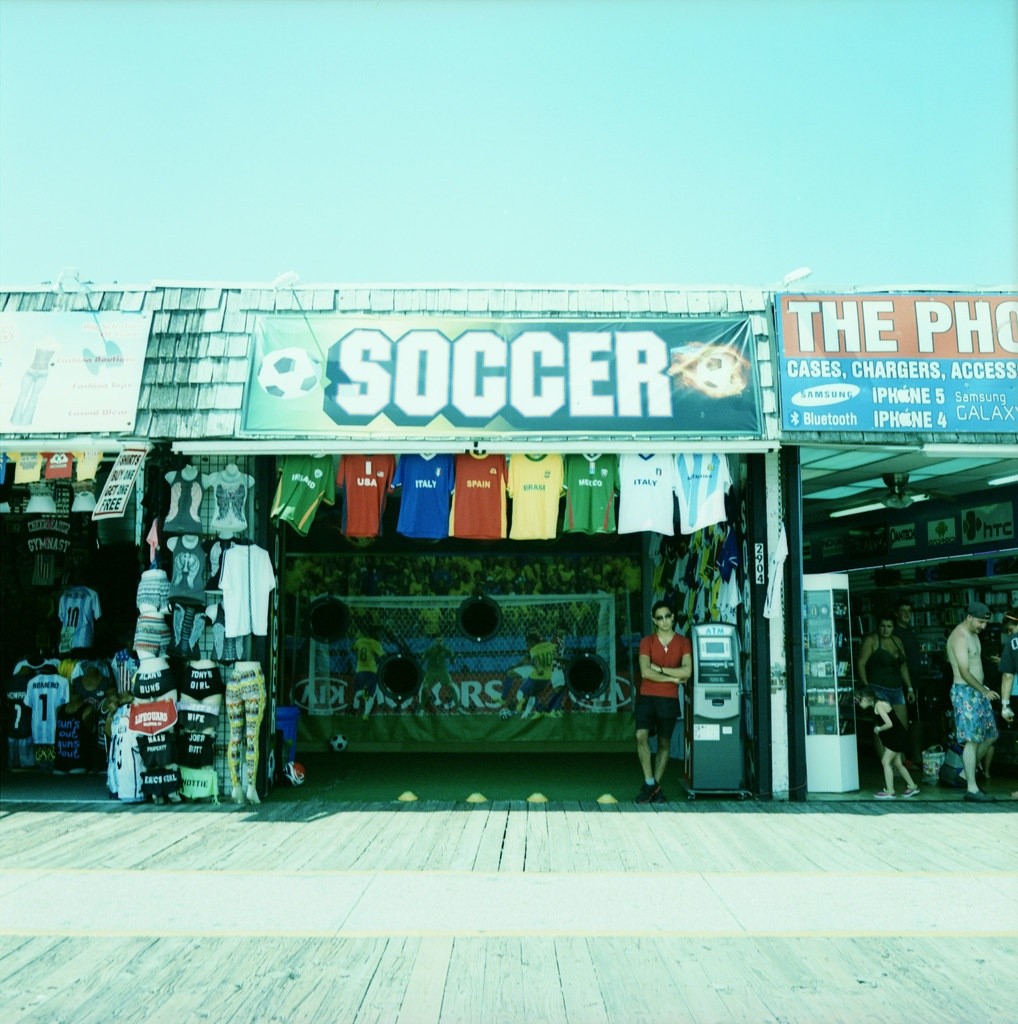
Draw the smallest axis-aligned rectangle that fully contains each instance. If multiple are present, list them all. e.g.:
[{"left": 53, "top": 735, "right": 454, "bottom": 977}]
[
  {"left": 956, "top": 776, "right": 986, "bottom": 793},
  {"left": 966, "top": 792, "right": 995, "bottom": 801}
]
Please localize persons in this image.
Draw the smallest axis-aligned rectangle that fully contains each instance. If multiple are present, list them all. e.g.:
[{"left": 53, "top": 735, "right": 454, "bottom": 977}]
[
  {"left": 946, "top": 602, "right": 1000, "bottom": 801},
  {"left": 1001, "top": 606, "right": 1018, "bottom": 729},
  {"left": 891, "top": 599, "right": 920, "bottom": 770},
  {"left": 853, "top": 684, "right": 921, "bottom": 798},
  {"left": 997, "top": 633, "right": 1018, "bottom": 721},
  {"left": 279, "top": 552, "right": 643, "bottom": 712},
  {"left": 634, "top": 600, "right": 693, "bottom": 804},
  {"left": 855, "top": 614, "right": 917, "bottom": 779},
  {"left": 2, "top": 462, "right": 269, "bottom": 806}
]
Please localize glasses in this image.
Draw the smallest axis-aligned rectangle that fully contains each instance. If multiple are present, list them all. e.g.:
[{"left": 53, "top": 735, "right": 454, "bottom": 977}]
[{"left": 656, "top": 614, "right": 671, "bottom": 620}]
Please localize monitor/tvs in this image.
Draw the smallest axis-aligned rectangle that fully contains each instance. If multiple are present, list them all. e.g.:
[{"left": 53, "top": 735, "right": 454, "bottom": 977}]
[{"left": 699, "top": 637, "right": 733, "bottom": 662}]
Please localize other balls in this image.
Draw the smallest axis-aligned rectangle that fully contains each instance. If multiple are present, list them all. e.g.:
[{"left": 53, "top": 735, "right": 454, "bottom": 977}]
[{"left": 283, "top": 762, "right": 306, "bottom": 786}]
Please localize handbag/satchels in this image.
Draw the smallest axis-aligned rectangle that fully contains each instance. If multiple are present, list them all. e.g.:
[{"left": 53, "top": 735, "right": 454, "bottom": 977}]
[
  {"left": 921, "top": 745, "right": 945, "bottom": 785},
  {"left": 940, "top": 746, "right": 970, "bottom": 788}
]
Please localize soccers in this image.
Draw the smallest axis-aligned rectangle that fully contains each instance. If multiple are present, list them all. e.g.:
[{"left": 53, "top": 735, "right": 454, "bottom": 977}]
[
  {"left": 329, "top": 733, "right": 348, "bottom": 752},
  {"left": 499, "top": 708, "right": 513, "bottom": 721}
]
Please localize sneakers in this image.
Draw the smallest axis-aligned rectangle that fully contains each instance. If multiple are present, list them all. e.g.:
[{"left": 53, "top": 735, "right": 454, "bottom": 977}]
[
  {"left": 635, "top": 780, "right": 659, "bottom": 802},
  {"left": 651, "top": 790, "right": 665, "bottom": 803},
  {"left": 873, "top": 792, "right": 896, "bottom": 798},
  {"left": 903, "top": 785, "right": 920, "bottom": 796}
]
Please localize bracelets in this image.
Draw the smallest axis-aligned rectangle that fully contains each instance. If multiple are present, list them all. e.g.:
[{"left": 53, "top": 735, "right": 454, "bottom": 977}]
[
  {"left": 877, "top": 727, "right": 881, "bottom": 732},
  {"left": 908, "top": 687, "right": 914, "bottom": 692},
  {"left": 1001, "top": 703, "right": 1010, "bottom": 708},
  {"left": 659, "top": 665, "right": 664, "bottom": 674}
]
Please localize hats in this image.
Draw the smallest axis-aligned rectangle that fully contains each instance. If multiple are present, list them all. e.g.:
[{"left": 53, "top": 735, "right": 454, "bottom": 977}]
[{"left": 968, "top": 602, "right": 991, "bottom": 618}]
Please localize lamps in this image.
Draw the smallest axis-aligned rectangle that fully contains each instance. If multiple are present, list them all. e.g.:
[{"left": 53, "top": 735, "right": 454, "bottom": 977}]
[{"left": 878, "top": 472, "right": 911, "bottom": 507}]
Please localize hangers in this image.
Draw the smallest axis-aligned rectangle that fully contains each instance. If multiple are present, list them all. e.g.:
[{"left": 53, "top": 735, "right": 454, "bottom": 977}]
[{"left": 238, "top": 535, "right": 253, "bottom": 545}]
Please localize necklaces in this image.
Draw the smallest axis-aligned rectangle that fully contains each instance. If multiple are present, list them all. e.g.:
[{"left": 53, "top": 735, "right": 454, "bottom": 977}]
[{"left": 656, "top": 629, "right": 674, "bottom": 653}]
